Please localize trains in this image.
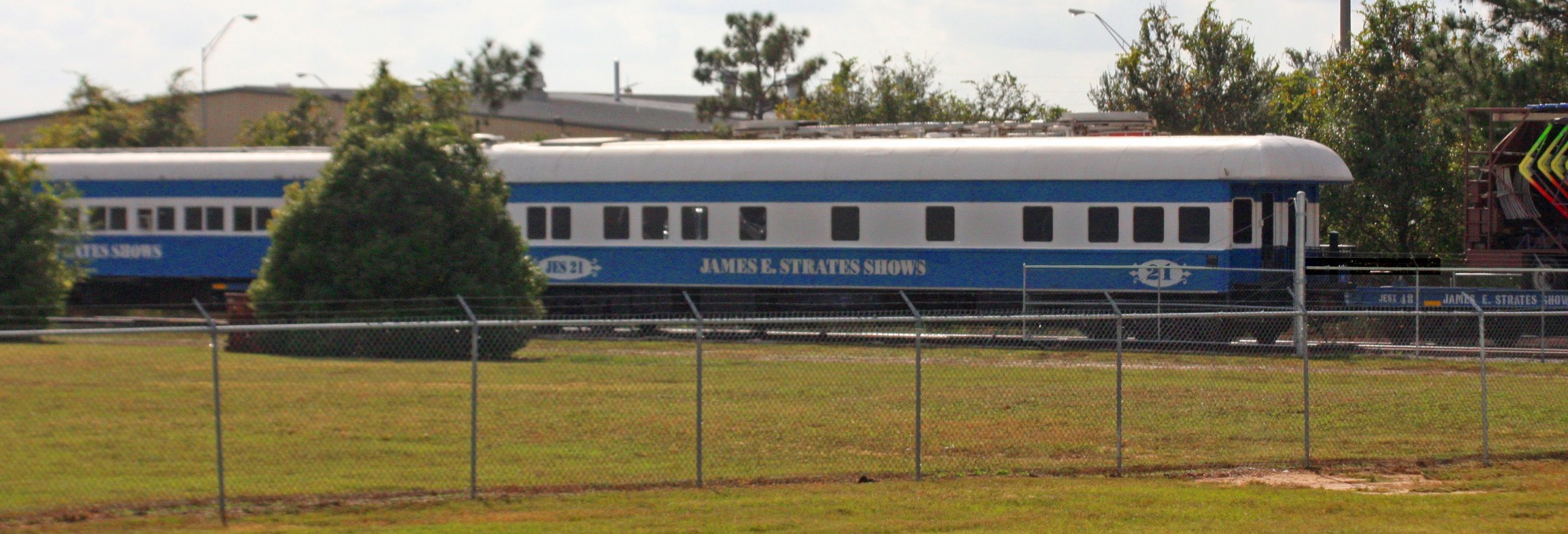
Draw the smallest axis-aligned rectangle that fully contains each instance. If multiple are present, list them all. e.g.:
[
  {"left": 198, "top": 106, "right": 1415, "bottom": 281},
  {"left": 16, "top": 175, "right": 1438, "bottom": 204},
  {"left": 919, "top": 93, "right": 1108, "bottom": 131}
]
[{"left": 2, "top": 134, "right": 1358, "bottom": 343}]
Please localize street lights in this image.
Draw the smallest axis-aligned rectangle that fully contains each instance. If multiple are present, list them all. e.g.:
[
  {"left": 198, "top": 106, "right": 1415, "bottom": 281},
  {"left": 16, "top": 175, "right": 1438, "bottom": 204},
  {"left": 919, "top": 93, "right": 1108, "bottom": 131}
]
[{"left": 199, "top": 14, "right": 261, "bottom": 147}]
